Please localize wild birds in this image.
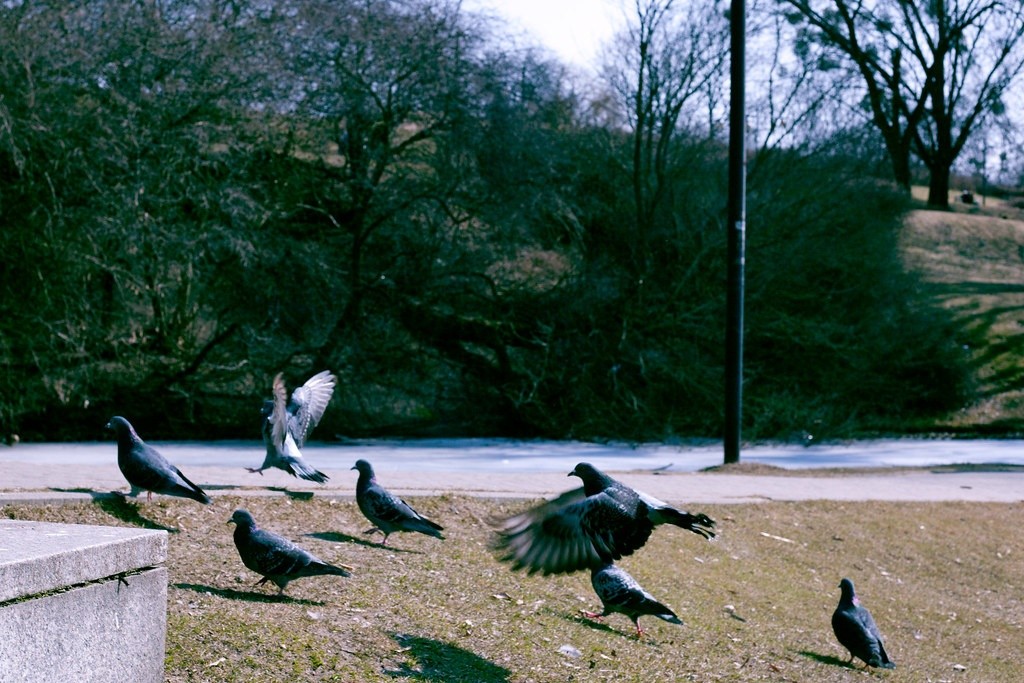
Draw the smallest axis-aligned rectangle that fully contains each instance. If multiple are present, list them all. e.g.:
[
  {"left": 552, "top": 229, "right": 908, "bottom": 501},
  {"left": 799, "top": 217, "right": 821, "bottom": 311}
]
[
  {"left": 243, "top": 370, "right": 335, "bottom": 487},
  {"left": 105, "top": 416, "right": 215, "bottom": 507},
  {"left": 830, "top": 577, "right": 895, "bottom": 672},
  {"left": 578, "top": 562, "right": 684, "bottom": 637},
  {"left": 227, "top": 509, "right": 352, "bottom": 598},
  {"left": 483, "top": 462, "right": 717, "bottom": 578},
  {"left": 350, "top": 459, "right": 446, "bottom": 545}
]
